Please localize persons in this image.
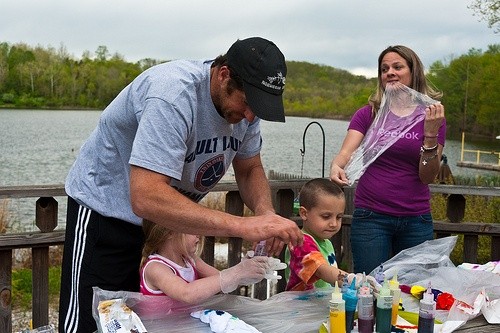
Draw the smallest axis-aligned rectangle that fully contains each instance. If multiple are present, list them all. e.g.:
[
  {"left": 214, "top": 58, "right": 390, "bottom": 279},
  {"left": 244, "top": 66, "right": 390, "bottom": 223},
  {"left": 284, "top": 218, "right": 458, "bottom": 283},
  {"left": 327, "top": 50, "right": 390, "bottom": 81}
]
[
  {"left": 138, "top": 219, "right": 288, "bottom": 317},
  {"left": 57, "top": 37, "right": 304, "bottom": 333},
  {"left": 330, "top": 45, "right": 447, "bottom": 276},
  {"left": 285, "top": 178, "right": 383, "bottom": 296}
]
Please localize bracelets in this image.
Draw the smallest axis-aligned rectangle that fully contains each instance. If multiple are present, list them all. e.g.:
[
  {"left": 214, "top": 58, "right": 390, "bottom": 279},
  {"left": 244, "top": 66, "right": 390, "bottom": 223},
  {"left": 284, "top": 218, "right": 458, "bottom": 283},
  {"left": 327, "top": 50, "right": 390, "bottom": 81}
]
[
  {"left": 424, "top": 133, "right": 440, "bottom": 140},
  {"left": 420, "top": 145, "right": 438, "bottom": 165}
]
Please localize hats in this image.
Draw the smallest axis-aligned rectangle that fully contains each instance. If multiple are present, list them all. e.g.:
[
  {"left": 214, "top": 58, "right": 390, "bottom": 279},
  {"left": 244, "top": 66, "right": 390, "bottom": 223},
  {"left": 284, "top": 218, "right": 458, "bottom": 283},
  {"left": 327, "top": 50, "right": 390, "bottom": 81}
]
[{"left": 227, "top": 37, "right": 287, "bottom": 123}]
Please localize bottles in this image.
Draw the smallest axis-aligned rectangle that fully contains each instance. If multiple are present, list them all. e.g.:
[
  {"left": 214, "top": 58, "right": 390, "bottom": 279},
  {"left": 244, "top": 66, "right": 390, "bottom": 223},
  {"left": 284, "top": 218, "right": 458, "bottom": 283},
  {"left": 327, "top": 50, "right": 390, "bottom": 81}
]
[
  {"left": 329, "top": 281, "right": 346, "bottom": 333},
  {"left": 418, "top": 281, "right": 436, "bottom": 333},
  {"left": 358, "top": 271, "right": 401, "bottom": 333},
  {"left": 342, "top": 277, "right": 358, "bottom": 333},
  {"left": 341, "top": 278, "right": 348, "bottom": 300},
  {"left": 254, "top": 240, "right": 267, "bottom": 256}
]
